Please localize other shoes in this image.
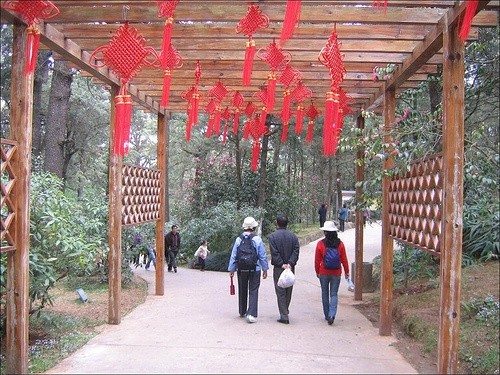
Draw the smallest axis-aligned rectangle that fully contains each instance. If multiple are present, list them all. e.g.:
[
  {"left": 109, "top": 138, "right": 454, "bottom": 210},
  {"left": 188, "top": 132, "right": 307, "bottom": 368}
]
[
  {"left": 241, "top": 310, "right": 248, "bottom": 317},
  {"left": 247, "top": 315, "right": 256, "bottom": 323},
  {"left": 325, "top": 315, "right": 328, "bottom": 320},
  {"left": 277, "top": 319, "right": 289, "bottom": 323},
  {"left": 328, "top": 316, "right": 334, "bottom": 325}
]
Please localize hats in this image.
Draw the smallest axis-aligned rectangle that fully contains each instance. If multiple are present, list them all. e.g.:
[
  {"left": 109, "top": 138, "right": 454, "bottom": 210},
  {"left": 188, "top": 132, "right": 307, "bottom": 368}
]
[
  {"left": 320, "top": 221, "right": 338, "bottom": 231},
  {"left": 242, "top": 217, "right": 258, "bottom": 230}
]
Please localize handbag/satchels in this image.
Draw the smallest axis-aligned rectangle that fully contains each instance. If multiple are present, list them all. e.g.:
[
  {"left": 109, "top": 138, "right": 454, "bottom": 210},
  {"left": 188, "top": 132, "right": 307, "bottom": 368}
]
[
  {"left": 230, "top": 276, "right": 235, "bottom": 295},
  {"left": 277, "top": 268, "right": 296, "bottom": 288},
  {"left": 347, "top": 278, "right": 356, "bottom": 292}
]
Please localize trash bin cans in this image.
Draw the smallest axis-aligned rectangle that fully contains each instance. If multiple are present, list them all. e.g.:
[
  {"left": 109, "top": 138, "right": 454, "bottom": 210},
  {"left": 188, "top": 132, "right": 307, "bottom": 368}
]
[{"left": 351, "top": 261, "right": 372, "bottom": 291}]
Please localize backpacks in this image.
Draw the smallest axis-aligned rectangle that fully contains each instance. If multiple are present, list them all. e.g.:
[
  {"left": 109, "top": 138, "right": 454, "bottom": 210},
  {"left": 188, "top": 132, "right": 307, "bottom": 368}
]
[
  {"left": 322, "top": 238, "right": 341, "bottom": 269},
  {"left": 233, "top": 233, "right": 259, "bottom": 276}
]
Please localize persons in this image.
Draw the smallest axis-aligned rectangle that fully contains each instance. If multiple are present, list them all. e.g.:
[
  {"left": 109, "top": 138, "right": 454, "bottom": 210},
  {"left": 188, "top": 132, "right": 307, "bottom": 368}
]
[
  {"left": 165, "top": 225, "right": 181, "bottom": 273},
  {"left": 130, "top": 234, "right": 156, "bottom": 269},
  {"left": 315, "top": 221, "right": 350, "bottom": 325},
  {"left": 194, "top": 240, "right": 210, "bottom": 271},
  {"left": 363, "top": 206, "right": 370, "bottom": 227},
  {"left": 269, "top": 215, "right": 299, "bottom": 324},
  {"left": 318, "top": 204, "right": 327, "bottom": 227},
  {"left": 338, "top": 204, "right": 348, "bottom": 232},
  {"left": 228, "top": 217, "right": 269, "bottom": 322}
]
